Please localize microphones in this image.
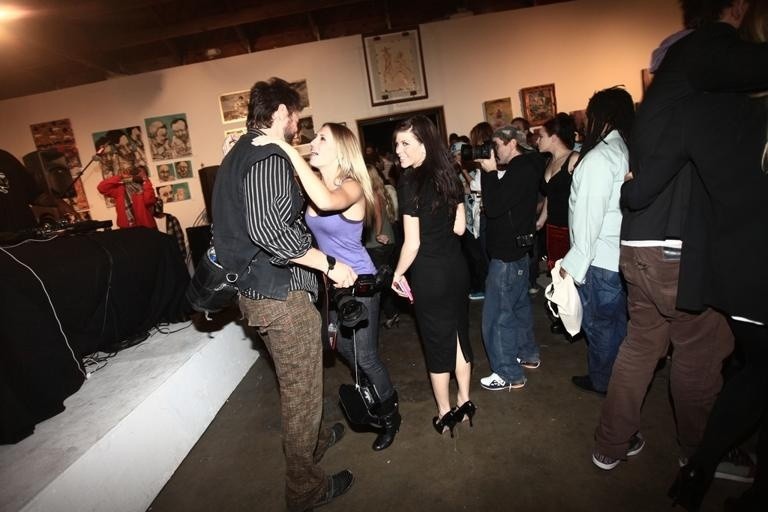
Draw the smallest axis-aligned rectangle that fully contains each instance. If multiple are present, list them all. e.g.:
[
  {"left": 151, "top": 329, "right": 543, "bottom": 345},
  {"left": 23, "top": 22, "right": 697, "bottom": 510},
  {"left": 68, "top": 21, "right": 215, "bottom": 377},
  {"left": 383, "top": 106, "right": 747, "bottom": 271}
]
[{"left": 93, "top": 145, "right": 105, "bottom": 161}]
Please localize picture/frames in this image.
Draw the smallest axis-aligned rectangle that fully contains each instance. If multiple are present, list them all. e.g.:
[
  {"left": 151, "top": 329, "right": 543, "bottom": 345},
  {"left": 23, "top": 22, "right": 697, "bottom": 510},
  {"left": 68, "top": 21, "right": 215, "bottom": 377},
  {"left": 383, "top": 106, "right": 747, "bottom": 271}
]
[
  {"left": 291, "top": 77, "right": 311, "bottom": 111},
  {"left": 484, "top": 96, "right": 513, "bottom": 127},
  {"left": 355, "top": 104, "right": 449, "bottom": 164},
  {"left": 361, "top": 23, "right": 429, "bottom": 106},
  {"left": 218, "top": 88, "right": 253, "bottom": 123},
  {"left": 518, "top": 82, "right": 558, "bottom": 130},
  {"left": 223, "top": 129, "right": 248, "bottom": 150},
  {"left": 294, "top": 116, "right": 316, "bottom": 147}
]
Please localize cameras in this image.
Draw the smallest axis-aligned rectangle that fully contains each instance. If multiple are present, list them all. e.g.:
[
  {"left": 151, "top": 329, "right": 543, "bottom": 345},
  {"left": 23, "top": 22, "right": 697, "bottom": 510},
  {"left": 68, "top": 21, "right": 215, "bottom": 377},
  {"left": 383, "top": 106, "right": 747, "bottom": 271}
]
[
  {"left": 461, "top": 141, "right": 497, "bottom": 161},
  {"left": 327, "top": 274, "right": 377, "bottom": 327}
]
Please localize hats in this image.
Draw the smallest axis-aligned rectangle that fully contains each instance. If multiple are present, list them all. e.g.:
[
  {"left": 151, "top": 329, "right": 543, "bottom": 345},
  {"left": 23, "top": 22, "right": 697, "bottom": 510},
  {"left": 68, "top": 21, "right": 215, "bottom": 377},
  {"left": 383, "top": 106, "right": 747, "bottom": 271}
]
[
  {"left": 490, "top": 125, "right": 536, "bottom": 151},
  {"left": 449, "top": 141, "right": 467, "bottom": 155}
]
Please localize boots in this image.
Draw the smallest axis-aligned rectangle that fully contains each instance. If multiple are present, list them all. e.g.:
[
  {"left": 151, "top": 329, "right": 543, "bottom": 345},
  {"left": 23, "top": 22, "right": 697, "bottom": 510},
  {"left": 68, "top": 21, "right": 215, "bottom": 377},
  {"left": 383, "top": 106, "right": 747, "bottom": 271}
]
[{"left": 372, "top": 389, "right": 402, "bottom": 451}]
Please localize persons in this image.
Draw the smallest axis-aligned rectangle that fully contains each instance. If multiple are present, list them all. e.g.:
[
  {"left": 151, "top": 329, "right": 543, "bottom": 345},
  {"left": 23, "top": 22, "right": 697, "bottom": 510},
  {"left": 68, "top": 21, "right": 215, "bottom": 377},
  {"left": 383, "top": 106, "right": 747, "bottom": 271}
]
[
  {"left": 590, "top": 2, "right": 768, "bottom": 512},
  {"left": 364, "top": 87, "right": 635, "bottom": 437},
  {"left": 1, "top": 150, "right": 81, "bottom": 244},
  {"left": 97, "top": 165, "right": 158, "bottom": 231},
  {"left": 211, "top": 77, "right": 403, "bottom": 510}
]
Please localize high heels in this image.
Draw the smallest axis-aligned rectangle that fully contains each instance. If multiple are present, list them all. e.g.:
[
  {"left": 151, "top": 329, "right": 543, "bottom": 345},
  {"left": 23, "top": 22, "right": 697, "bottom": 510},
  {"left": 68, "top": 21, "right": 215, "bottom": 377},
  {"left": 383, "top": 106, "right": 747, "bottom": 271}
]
[
  {"left": 384, "top": 314, "right": 401, "bottom": 328},
  {"left": 451, "top": 400, "right": 476, "bottom": 428},
  {"left": 432, "top": 410, "right": 457, "bottom": 439}
]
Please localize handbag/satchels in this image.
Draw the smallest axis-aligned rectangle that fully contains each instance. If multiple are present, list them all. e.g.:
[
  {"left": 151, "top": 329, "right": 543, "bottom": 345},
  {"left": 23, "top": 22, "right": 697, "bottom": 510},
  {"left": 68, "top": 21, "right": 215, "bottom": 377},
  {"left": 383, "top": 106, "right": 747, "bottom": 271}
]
[
  {"left": 544, "top": 256, "right": 585, "bottom": 340},
  {"left": 337, "top": 381, "right": 387, "bottom": 434},
  {"left": 452, "top": 229, "right": 488, "bottom": 286},
  {"left": 184, "top": 244, "right": 241, "bottom": 315}
]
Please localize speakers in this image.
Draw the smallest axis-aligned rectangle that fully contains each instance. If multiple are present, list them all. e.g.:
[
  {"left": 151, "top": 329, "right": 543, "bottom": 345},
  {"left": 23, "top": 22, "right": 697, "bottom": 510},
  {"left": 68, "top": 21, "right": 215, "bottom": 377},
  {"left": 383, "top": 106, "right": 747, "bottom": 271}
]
[
  {"left": 198, "top": 165, "right": 220, "bottom": 224},
  {"left": 186, "top": 225, "right": 211, "bottom": 273},
  {"left": 22, "top": 150, "right": 78, "bottom": 206}
]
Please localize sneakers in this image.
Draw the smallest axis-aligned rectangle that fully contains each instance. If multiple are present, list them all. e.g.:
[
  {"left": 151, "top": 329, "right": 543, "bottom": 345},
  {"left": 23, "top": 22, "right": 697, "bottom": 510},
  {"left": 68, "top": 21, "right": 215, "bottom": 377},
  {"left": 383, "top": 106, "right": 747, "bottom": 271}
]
[
  {"left": 468, "top": 291, "right": 489, "bottom": 301},
  {"left": 548, "top": 318, "right": 567, "bottom": 335},
  {"left": 572, "top": 374, "right": 609, "bottom": 397},
  {"left": 516, "top": 356, "right": 541, "bottom": 369},
  {"left": 328, "top": 422, "right": 347, "bottom": 446},
  {"left": 591, "top": 429, "right": 648, "bottom": 471},
  {"left": 479, "top": 370, "right": 528, "bottom": 391},
  {"left": 310, "top": 469, "right": 355, "bottom": 507},
  {"left": 679, "top": 442, "right": 758, "bottom": 483}
]
